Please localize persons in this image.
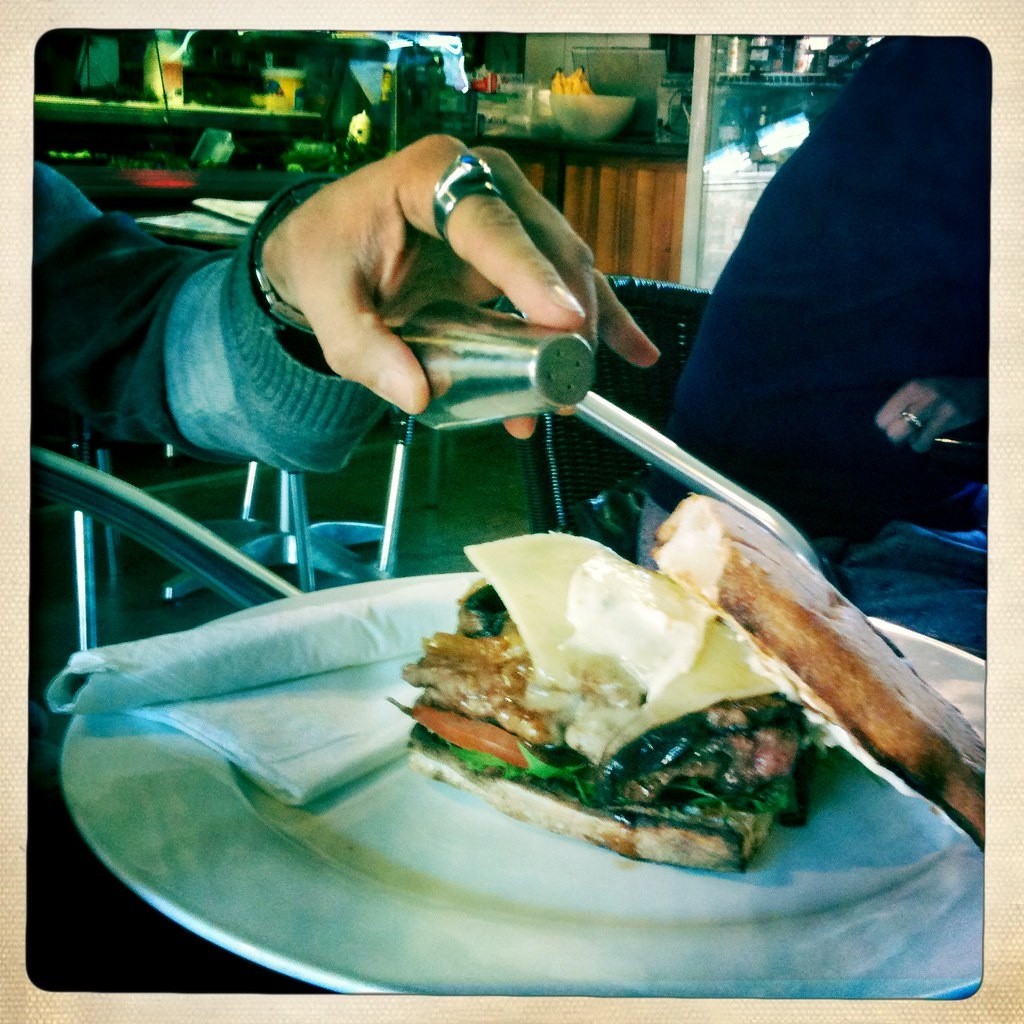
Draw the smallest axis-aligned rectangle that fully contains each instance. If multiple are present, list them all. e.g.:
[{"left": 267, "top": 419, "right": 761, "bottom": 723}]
[
  {"left": 31, "top": 133, "right": 663, "bottom": 475},
  {"left": 639, "top": 32, "right": 994, "bottom": 661}
]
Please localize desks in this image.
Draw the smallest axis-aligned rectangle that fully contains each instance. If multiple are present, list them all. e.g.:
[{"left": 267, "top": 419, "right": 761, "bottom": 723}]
[{"left": 135, "top": 216, "right": 392, "bottom": 599}]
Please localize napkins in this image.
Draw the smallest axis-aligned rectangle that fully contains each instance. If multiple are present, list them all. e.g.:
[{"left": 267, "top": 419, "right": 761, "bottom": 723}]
[{"left": 44, "top": 573, "right": 481, "bottom": 809}]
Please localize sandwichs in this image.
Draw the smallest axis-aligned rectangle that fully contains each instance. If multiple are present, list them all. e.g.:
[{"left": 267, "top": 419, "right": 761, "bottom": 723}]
[{"left": 388, "top": 493, "right": 984, "bottom": 870}]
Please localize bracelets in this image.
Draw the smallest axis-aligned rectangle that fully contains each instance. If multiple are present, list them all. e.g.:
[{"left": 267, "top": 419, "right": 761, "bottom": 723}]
[{"left": 250, "top": 180, "right": 335, "bottom": 335}]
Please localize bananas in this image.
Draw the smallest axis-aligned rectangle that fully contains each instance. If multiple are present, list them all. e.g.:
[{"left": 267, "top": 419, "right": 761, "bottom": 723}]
[{"left": 551, "top": 65, "right": 595, "bottom": 95}]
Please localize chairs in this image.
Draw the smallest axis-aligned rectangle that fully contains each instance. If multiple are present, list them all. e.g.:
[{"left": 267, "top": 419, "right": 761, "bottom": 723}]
[{"left": 497, "top": 277, "right": 714, "bottom": 536}]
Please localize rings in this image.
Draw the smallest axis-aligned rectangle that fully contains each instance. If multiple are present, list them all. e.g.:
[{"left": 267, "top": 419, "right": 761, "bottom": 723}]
[
  {"left": 430, "top": 153, "right": 501, "bottom": 243},
  {"left": 900, "top": 408, "right": 923, "bottom": 430}
]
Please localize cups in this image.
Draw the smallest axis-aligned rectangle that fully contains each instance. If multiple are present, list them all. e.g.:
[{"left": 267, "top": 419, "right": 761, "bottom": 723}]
[{"left": 262, "top": 70, "right": 308, "bottom": 113}]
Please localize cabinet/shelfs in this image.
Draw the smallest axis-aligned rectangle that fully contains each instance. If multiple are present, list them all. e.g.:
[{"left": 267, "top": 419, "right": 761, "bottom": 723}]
[{"left": 680, "top": 34, "right": 884, "bottom": 288}]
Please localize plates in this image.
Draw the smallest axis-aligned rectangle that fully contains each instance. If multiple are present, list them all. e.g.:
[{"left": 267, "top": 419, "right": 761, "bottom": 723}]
[{"left": 58, "top": 572, "right": 987, "bottom": 995}]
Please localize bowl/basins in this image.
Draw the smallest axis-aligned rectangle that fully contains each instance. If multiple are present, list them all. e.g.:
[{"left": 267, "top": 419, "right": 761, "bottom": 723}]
[
  {"left": 190, "top": 128, "right": 235, "bottom": 167},
  {"left": 549, "top": 95, "right": 636, "bottom": 142}
]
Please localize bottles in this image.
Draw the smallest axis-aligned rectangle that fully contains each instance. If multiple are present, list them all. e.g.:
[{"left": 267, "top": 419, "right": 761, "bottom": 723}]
[
  {"left": 380, "top": 291, "right": 596, "bottom": 429},
  {"left": 825, "top": 34, "right": 851, "bottom": 72},
  {"left": 750, "top": 105, "right": 766, "bottom": 163},
  {"left": 740, "top": 105, "right": 754, "bottom": 152},
  {"left": 717, "top": 98, "right": 743, "bottom": 148}
]
